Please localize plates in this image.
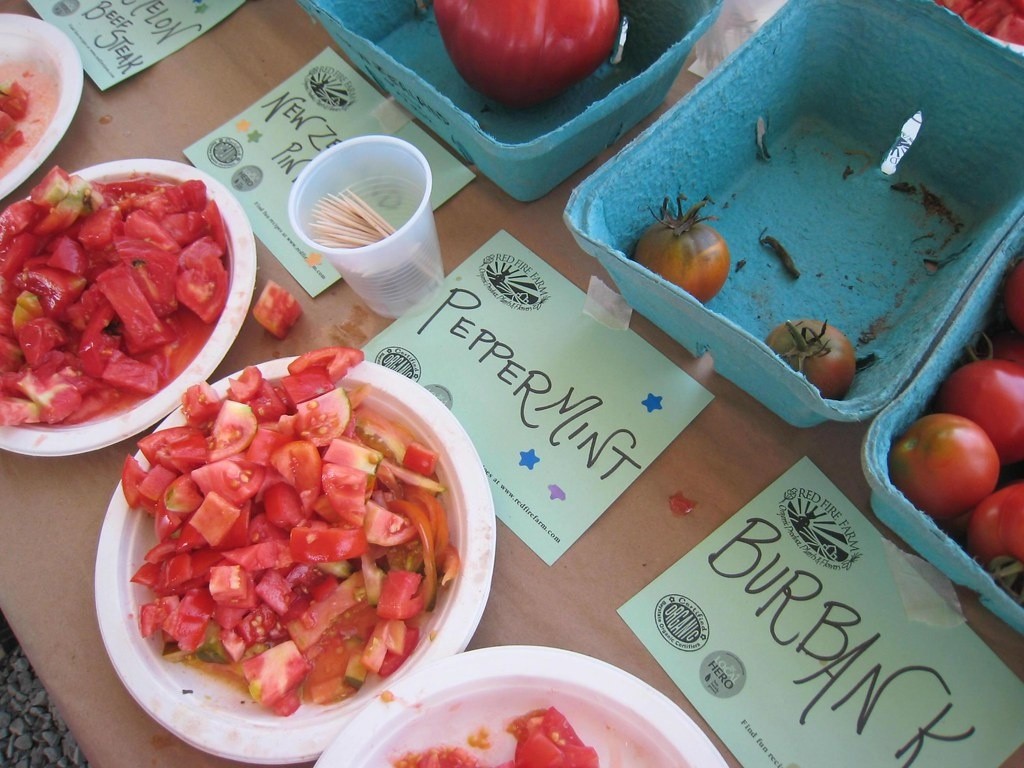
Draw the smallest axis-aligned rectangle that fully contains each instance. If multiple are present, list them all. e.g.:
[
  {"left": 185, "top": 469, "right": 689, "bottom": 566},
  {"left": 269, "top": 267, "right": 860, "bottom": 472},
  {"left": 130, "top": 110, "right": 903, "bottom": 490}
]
[
  {"left": 0, "top": 12, "right": 85, "bottom": 203},
  {"left": 95, "top": 355, "right": 497, "bottom": 766},
  {"left": 312, "top": 645, "right": 730, "bottom": 768},
  {"left": 0, "top": 159, "right": 258, "bottom": 456}
]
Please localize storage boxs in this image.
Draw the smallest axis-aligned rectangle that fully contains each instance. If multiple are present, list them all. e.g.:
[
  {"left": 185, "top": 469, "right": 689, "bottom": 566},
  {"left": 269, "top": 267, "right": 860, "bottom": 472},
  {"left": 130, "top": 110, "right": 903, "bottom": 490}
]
[
  {"left": 294, "top": 0, "right": 723, "bottom": 205},
  {"left": 859, "top": 219, "right": 1024, "bottom": 633},
  {"left": 563, "top": 0, "right": 1024, "bottom": 430}
]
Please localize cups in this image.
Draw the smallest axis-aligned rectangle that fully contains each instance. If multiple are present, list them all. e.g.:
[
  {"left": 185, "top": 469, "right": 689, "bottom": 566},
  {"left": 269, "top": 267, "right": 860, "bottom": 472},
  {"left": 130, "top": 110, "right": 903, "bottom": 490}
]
[{"left": 286, "top": 134, "right": 445, "bottom": 318}]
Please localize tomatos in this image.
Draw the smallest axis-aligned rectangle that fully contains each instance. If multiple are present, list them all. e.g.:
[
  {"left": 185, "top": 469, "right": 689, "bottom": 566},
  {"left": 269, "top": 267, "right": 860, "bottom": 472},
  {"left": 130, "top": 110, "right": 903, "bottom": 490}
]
[
  {"left": 120, "top": 346, "right": 602, "bottom": 767},
  {"left": 433, "top": 0, "right": 620, "bottom": 107},
  {"left": 933, "top": 0, "right": 1024, "bottom": 48},
  {"left": 0, "top": 78, "right": 300, "bottom": 429},
  {"left": 888, "top": 256, "right": 1024, "bottom": 608},
  {"left": 635, "top": 194, "right": 730, "bottom": 304},
  {"left": 764, "top": 319, "right": 855, "bottom": 397}
]
